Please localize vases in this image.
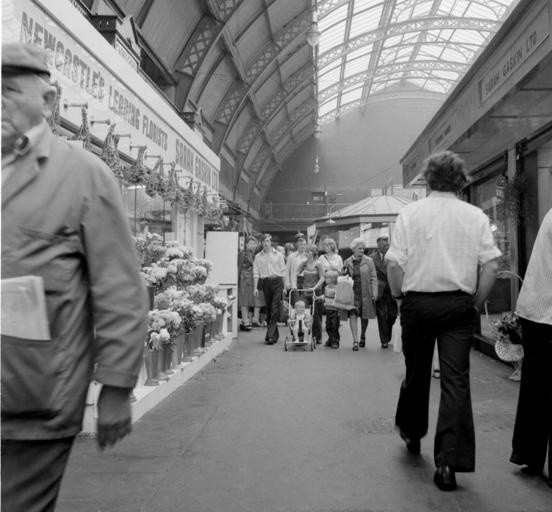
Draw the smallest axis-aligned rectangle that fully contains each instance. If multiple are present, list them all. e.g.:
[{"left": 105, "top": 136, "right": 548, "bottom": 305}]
[{"left": 144, "top": 317, "right": 223, "bottom": 386}]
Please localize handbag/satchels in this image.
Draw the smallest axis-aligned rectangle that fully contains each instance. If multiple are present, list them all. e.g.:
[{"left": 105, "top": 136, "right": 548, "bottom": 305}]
[
  {"left": 277, "top": 294, "right": 289, "bottom": 322},
  {"left": 332, "top": 274, "right": 354, "bottom": 311}
]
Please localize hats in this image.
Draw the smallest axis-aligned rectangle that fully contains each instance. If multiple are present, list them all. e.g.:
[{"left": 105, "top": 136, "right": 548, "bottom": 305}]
[{"left": 1, "top": 42, "right": 51, "bottom": 77}]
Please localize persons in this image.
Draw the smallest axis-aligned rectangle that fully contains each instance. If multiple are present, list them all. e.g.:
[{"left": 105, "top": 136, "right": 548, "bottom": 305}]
[
  {"left": 369, "top": 236, "right": 398, "bottom": 350},
  {"left": 385, "top": 150, "right": 503, "bottom": 493},
  {"left": 2, "top": 43, "right": 151, "bottom": 510},
  {"left": 339, "top": 237, "right": 378, "bottom": 354},
  {"left": 237, "top": 233, "right": 347, "bottom": 349},
  {"left": 508, "top": 209, "right": 552, "bottom": 488}
]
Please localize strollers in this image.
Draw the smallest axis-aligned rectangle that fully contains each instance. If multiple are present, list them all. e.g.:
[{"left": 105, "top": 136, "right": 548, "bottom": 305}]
[{"left": 284, "top": 288, "right": 316, "bottom": 351}]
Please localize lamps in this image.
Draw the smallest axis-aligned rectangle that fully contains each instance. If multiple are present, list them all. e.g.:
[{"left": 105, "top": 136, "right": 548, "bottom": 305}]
[
  {"left": 306, "top": 6, "right": 321, "bottom": 46},
  {"left": 312, "top": 115, "right": 323, "bottom": 175}
]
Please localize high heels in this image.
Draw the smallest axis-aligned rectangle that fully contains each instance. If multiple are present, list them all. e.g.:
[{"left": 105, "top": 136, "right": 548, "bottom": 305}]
[{"left": 240, "top": 321, "right": 251, "bottom": 331}]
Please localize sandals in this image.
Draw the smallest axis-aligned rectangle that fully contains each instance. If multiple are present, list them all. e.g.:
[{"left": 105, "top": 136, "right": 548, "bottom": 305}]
[
  {"left": 353, "top": 341, "right": 359, "bottom": 350},
  {"left": 359, "top": 336, "right": 365, "bottom": 347}
]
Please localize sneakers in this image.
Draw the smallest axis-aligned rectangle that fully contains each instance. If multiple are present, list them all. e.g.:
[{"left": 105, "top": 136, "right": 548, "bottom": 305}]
[{"left": 382, "top": 342, "right": 388, "bottom": 348}]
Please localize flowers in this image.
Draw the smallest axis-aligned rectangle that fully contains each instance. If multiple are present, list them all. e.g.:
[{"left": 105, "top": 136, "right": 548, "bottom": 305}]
[
  {"left": 130, "top": 232, "right": 225, "bottom": 346},
  {"left": 68, "top": 106, "right": 224, "bottom": 224}
]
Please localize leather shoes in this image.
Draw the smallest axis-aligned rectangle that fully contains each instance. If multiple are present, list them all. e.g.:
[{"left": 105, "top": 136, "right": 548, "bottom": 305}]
[
  {"left": 399, "top": 430, "right": 421, "bottom": 454},
  {"left": 434, "top": 465, "right": 458, "bottom": 491}
]
